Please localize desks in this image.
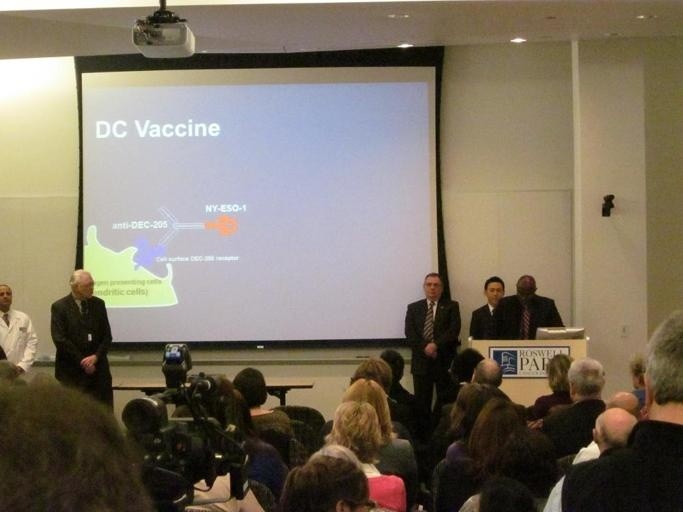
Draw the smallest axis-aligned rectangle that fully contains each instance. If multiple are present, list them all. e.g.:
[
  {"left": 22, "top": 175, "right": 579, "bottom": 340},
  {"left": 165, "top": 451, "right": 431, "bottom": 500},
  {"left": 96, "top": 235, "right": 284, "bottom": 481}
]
[{"left": 112, "top": 376, "right": 315, "bottom": 408}]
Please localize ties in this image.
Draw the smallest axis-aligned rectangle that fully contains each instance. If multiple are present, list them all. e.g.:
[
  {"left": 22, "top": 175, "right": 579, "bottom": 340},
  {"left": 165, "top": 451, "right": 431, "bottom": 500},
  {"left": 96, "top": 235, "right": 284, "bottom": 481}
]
[
  {"left": 519, "top": 304, "right": 532, "bottom": 341},
  {"left": 2, "top": 311, "right": 11, "bottom": 329},
  {"left": 422, "top": 300, "right": 436, "bottom": 344},
  {"left": 492, "top": 308, "right": 497, "bottom": 317},
  {"left": 80, "top": 299, "right": 89, "bottom": 322}
]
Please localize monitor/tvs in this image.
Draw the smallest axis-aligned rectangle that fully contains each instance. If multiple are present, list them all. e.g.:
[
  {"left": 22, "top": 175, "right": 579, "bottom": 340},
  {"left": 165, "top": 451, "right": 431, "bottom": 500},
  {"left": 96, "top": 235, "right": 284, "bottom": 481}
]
[{"left": 534, "top": 326, "right": 585, "bottom": 339}]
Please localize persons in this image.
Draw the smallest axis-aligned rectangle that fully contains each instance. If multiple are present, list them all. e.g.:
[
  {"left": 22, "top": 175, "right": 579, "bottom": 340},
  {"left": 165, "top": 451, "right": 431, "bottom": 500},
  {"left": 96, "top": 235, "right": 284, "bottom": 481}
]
[
  {"left": 0, "top": 267, "right": 152, "bottom": 511},
  {"left": 544, "top": 310, "right": 683, "bottom": 512},
  {"left": 186, "top": 349, "right": 420, "bottom": 511},
  {"left": 402, "top": 272, "right": 606, "bottom": 512}
]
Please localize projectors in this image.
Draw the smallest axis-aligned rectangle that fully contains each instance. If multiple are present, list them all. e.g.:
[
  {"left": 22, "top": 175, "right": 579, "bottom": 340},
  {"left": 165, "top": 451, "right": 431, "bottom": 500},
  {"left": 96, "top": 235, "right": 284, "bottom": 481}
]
[{"left": 131, "top": 20, "right": 196, "bottom": 61}]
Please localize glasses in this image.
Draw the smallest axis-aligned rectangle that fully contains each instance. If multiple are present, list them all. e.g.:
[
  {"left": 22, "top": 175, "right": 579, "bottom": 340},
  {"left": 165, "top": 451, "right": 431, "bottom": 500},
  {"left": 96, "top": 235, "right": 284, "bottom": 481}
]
[{"left": 343, "top": 498, "right": 379, "bottom": 512}]
